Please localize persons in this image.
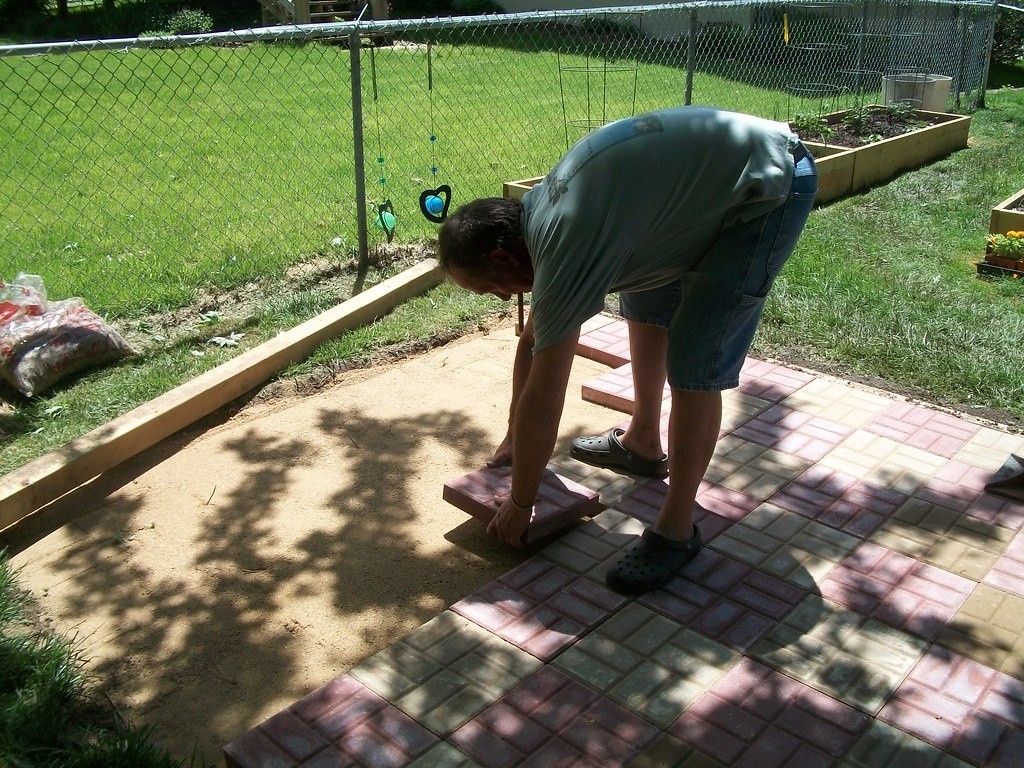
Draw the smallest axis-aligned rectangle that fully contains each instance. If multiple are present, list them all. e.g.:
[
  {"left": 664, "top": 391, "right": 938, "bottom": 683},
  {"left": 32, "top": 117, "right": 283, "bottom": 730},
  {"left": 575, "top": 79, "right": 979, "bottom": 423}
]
[{"left": 438, "top": 107, "right": 820, "bottom": 594}]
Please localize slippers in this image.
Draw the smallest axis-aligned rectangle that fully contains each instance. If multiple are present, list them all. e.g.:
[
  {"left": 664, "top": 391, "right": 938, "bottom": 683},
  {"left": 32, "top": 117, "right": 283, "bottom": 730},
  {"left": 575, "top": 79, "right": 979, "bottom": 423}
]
[
  {"left": 606, "top": 524, "right": 702, "bottom": 595},
  {"left": 570, "top": 428, "right": 669, "bottom": 479}
]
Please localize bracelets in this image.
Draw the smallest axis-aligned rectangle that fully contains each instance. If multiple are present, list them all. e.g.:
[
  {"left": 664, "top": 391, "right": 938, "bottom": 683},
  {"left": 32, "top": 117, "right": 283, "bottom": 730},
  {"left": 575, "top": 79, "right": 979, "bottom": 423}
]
[{"left": 511, "top": 493, "right": 533, "bottom": 508}]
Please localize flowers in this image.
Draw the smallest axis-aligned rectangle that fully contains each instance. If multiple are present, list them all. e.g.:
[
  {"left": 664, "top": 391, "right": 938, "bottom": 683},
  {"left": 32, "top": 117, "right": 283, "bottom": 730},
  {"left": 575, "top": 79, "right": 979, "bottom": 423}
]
[{"left": 986, "top": 230, "right": 1024, "bottom": 260}]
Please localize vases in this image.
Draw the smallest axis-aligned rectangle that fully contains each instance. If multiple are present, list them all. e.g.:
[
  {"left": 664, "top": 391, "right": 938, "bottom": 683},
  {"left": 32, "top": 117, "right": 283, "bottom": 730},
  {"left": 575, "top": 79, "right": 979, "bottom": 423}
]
[{"left": 987, "top": 255, "right": 1024, "bottom": 272}]
